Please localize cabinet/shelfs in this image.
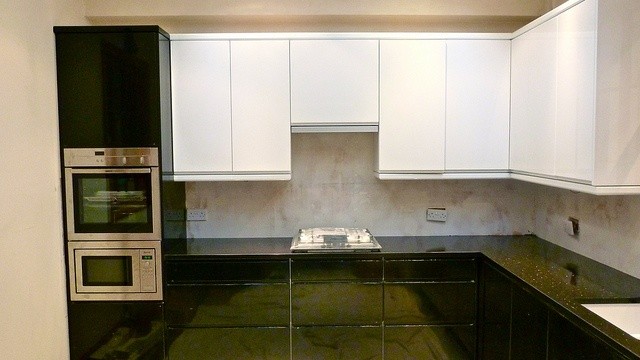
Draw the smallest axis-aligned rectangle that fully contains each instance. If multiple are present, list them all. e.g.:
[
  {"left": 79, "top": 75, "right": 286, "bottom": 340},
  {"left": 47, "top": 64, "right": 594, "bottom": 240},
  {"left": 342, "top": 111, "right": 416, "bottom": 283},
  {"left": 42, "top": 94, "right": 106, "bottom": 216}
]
[
  {"left": 171, "top": 34, "right": 291, "bottom": 180},
  {"left": 378, "top": 32, "right": 509, "bottom": 181},
  {"left": 382, "top": 252, "right": 480, "bottom": 359},
  {"left": 289, "top": 32, "right": 378, "bottom": 132},
  {"left": 480, "top": 262, "right": 550, "bottom": 359},
  {"left": 162, "top": 258, "right": 292, "bottom": 358},
  {"left": 552, "top": 307, "right": 629, "bottom": 359},
  {"left": 292, "top": 254, "right": 384, "bottom": 360},
  {"left": 509, "top": 1, "right": 600, "bottom": 194}
]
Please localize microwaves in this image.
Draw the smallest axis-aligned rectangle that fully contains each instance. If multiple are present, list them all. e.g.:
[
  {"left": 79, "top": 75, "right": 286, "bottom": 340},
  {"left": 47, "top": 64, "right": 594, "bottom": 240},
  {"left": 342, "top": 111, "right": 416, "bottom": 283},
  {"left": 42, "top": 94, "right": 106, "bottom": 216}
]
[{"left": 73, "top": 248, "right": 158, "bottom": 295}]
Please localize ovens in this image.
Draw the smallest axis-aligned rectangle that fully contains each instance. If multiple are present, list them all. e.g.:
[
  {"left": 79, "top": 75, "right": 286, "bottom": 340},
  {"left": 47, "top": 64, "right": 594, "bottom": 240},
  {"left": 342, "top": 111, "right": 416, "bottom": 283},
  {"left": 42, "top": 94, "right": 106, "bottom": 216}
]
[{"left": 62, "top": 147, "right": 164, "bottom": 241}]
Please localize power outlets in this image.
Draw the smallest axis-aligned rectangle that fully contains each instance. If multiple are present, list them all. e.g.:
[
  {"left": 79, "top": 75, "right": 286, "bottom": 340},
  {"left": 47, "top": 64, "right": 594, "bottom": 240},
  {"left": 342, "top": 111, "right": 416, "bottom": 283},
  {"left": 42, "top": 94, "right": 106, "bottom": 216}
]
[
  {"left": 164, "top": 207, "right": 185, "bottom": 221},
  {"left": 187, "top": 209, "right": 208, "bottom": 222},
  {"left": 563, "top": 220, "right": 575, "bottom": 236},
  {"left": 427, "top": 209, "right": 447, "bottom": 221}
]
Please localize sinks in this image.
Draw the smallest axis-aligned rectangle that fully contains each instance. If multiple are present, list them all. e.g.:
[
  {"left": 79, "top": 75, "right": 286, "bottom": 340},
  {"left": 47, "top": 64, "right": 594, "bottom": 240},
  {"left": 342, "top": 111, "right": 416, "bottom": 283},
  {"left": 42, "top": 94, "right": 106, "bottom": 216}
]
[{"left": 581, "top": 303, "right": 640, "bottom": 340}]
